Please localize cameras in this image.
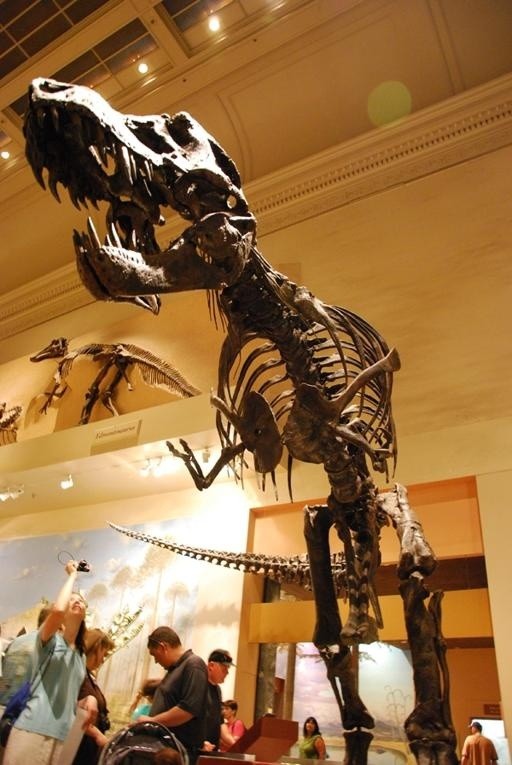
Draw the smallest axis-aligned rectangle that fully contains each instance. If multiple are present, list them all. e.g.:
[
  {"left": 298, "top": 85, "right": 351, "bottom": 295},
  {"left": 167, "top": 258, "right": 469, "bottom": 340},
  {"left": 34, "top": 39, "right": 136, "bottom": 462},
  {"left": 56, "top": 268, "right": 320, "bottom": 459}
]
[{"left": 73, "top": 560, "right": 90, "bottom": 572}]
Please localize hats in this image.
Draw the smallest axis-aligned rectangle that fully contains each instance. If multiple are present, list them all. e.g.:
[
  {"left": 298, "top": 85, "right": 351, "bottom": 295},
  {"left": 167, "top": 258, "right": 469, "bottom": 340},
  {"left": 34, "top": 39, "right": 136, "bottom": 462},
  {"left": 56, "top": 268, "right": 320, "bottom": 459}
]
[
  {"left": 468, "top": 722, "right": 481, "bottom": 727},
  {"left": 209, "top": 650, "right": 237, "bottom": 667}
]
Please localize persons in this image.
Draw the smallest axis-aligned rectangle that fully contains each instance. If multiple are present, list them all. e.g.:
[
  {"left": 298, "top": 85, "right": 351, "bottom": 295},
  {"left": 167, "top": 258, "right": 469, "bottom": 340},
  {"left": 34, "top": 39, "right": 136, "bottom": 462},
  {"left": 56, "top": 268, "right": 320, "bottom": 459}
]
[
  {"left": 457, "top": 721, "right": 498, "bottom": 765},
  {"left": 300, "top": 717, "right": 329, "bottom": 764},
  {"left": 128, "top": 626, "right": 246, "bottom": 765},
  {"left": 2, "top": 559, "right": 114, "bottom": 765}
]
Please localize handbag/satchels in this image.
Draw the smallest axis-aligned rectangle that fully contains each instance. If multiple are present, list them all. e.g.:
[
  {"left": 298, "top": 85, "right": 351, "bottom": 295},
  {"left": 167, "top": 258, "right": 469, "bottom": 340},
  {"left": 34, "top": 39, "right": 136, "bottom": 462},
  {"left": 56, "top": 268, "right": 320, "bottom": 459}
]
[{"left": 2, "top": 681, "right": 32, "bottom": 723}]
